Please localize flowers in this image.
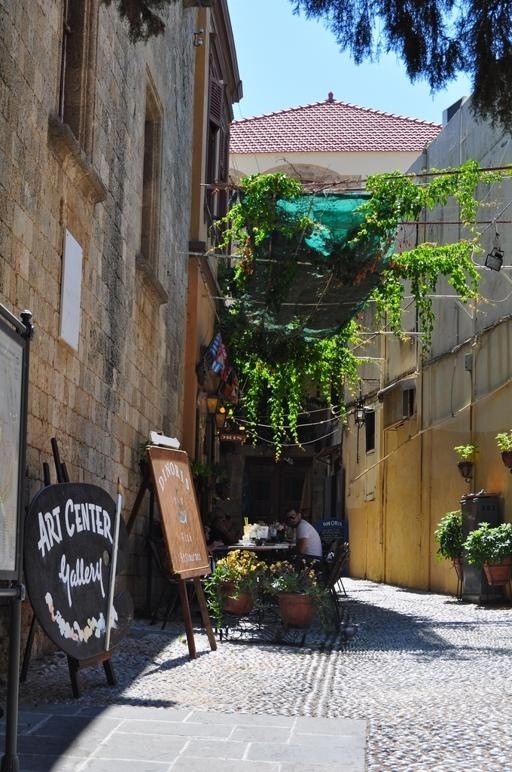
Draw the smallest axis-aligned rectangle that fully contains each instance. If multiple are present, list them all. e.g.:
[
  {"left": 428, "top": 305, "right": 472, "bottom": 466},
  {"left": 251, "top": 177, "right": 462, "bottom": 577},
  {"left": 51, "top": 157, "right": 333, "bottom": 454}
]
[{"left": 203, "top": 548, "right": 338, "bottom": 636}]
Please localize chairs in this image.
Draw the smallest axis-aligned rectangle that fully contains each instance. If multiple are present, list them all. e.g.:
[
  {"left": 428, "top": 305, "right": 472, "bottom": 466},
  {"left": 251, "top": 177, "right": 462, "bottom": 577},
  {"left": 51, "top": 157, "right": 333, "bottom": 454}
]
[
  {"left": 271, "top": 537, "right": 354, "bottom": 648},
  {"left": 143, "top": 538, "right": 211, "bottom": 629}
]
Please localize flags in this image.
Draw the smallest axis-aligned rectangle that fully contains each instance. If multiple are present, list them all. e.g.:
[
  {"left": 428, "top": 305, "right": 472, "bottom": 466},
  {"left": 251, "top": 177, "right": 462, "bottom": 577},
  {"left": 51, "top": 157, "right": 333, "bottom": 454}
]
[{"left": 206, "top": 328, "right": 239, "bottom": 406}]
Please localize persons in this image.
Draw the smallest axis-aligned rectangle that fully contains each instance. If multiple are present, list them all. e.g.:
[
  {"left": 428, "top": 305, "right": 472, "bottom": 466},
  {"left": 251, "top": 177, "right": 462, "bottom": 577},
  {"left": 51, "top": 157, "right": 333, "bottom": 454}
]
[
  {"left": 158, "top": 512, "right": 239, "bottom": 581},
  {"left": 283, "top": 508, "right": 323, "bottom": 571}
]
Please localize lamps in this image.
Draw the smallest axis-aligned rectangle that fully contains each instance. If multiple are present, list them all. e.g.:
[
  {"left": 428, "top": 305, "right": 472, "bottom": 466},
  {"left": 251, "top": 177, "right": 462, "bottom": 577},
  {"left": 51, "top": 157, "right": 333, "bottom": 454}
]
[
  {"left": 484, "top": 222, "right": 504, "bottom": 271},
  {"left": 353, "top": 378, "right": 367, "bottom": 429},
  {"left": 202, "top": 389, "right": 226, "bottom": 436}
]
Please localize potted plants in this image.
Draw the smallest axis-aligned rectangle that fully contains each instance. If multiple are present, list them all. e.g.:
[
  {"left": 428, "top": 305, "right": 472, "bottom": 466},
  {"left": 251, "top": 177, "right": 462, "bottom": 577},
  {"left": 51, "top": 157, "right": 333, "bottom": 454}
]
[
  {"left": 492, "top": 430, "right": 511, "bottom": 469},
  {"left": 435, "top": 510, "right": 511, "bottom": 587},
  {"left": 454, "top": 444, "right": 477, "bottom": 478}
]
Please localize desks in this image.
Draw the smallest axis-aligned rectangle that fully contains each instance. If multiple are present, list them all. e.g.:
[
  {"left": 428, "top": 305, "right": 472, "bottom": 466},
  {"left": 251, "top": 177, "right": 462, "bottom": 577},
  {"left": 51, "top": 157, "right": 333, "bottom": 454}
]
[{"left": 226, "top": 543, "right": 290, "bottom": 627}]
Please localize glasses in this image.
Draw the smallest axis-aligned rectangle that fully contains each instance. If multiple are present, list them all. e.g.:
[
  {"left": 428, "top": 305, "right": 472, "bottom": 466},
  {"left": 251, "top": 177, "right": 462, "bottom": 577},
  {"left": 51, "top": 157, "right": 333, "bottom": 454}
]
[{"left": 286, "top": 514, "right": 297, "bottom": 522}]
